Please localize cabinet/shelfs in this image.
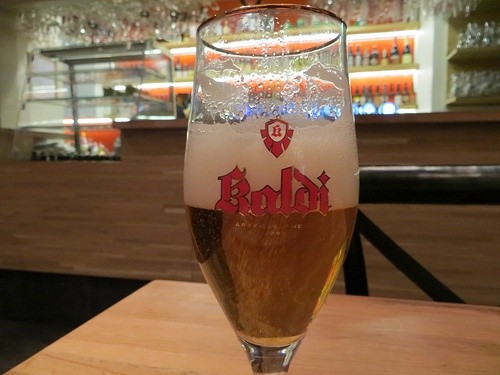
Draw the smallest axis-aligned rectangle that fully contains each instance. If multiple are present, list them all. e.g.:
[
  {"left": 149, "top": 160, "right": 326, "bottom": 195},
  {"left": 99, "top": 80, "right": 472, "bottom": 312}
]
[
  {"left": 17, "top": 41, "right": 176, "bottom": 128},
  {"left": 446, "top": 14, "right": 500, "bottom": 105},
  {"left": 105, "top": 23, "right": 418, "bottom": 110}
]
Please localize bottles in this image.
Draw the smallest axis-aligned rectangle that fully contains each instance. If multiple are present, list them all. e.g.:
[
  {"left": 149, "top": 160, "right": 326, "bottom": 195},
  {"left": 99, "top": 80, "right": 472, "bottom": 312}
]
[
  {"left": 346, "top": 37, "right": 412, "bottom": 66},
  {"left": 353, "top": 83, "right": 416, "bottom": 112},
  {"left": 175, "top": 56, "right": 193, "bottom": 80}
]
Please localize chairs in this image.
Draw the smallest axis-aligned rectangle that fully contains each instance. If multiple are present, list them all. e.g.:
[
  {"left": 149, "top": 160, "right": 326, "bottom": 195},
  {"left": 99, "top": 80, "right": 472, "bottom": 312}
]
[{"left": 344, "top": 163, "right": 500, "bottom": 304}]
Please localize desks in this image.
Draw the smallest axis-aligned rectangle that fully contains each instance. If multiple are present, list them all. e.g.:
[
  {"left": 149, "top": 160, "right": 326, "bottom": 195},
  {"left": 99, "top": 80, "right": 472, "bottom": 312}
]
[{"left": 3, "top": 278, "right": 500, "bottom": 375}]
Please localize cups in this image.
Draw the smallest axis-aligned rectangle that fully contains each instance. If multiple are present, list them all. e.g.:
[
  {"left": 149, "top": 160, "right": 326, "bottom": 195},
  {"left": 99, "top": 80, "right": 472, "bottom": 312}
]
[{"left": 182, "top": 4, "right": 360, "bottom": 375}]
